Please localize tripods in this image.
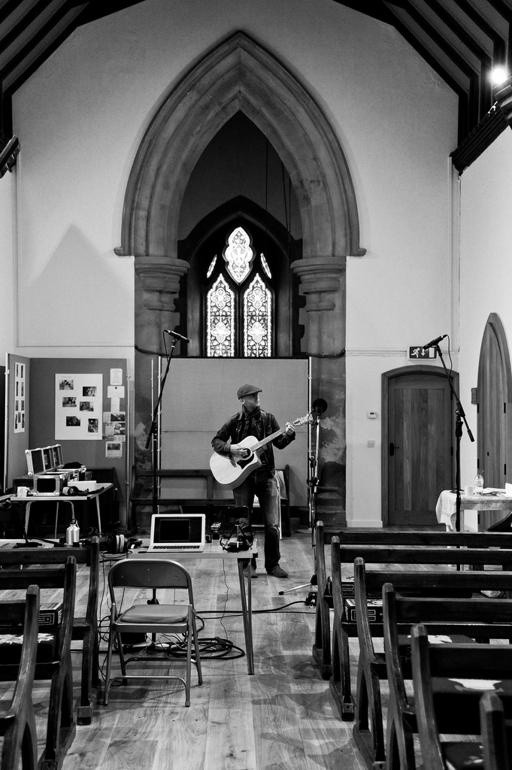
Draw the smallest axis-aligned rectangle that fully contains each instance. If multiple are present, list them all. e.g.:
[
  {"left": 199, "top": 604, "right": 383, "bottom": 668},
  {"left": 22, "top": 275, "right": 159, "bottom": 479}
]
[{"left": 278, "top": 420, "right": 321, "bottom": 596}]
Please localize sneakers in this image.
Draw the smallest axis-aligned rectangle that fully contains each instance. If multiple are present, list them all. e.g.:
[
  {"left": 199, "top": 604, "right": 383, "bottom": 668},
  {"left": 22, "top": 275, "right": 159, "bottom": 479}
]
[
  {"left": 267, "top": 565, "right": 288, "bottom": 578},
  {"left": 243, "top": 566, "right": 257, "bottom": 578}
]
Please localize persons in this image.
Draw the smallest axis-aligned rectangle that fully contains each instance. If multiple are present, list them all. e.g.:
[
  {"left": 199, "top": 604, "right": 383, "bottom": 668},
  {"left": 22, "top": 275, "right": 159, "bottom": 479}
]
[{"left": 211, "top": 384, "right": 296, "bottom": 579}]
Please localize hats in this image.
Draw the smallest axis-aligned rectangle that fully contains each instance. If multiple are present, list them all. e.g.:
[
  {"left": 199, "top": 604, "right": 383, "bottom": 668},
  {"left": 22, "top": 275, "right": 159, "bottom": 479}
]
[{"left": 237, "top": 384, "right": 262, "bottom": 399}]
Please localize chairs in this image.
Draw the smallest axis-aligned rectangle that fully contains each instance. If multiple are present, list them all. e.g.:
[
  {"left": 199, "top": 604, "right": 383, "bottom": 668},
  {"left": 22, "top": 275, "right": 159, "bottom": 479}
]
[{"left": 102, "top": 559, "right": 208, "bottom": 707}]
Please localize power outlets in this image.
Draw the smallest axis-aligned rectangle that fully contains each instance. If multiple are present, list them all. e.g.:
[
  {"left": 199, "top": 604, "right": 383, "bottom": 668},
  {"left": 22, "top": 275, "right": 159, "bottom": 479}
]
[{"left": 367, "top": 409, "right": 376, "bottom": 419}]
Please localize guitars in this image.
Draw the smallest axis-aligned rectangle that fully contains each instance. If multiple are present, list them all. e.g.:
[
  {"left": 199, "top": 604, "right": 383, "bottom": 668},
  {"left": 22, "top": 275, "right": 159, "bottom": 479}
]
[{"left": 209, "top": 413, "right": 312, "bottom": 484}]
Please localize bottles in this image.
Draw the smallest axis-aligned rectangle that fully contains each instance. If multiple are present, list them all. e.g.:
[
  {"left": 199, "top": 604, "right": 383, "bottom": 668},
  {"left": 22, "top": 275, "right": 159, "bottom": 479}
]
[{"left": 66, "top": 522, "right": 80, "bottom": 546}]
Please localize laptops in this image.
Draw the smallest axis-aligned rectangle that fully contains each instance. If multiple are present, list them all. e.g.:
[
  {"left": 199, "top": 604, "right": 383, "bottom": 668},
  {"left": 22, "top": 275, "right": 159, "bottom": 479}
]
[{"left": 147, "top": 513, "right": 206, "bottom": 554}]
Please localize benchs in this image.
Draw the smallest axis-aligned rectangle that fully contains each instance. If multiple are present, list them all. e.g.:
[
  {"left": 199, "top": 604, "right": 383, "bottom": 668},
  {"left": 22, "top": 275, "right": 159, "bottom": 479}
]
[
  {"left": 408, "top": 622, "right": 509, "bottom": 764},
  {"left": 381, "top": 581, "right": 512, "bottom": 761},
  {"left": 0, "top": 546, "right": 103, "bottom": 723},
  {"left": 2, "top": 551, "right": 78, "bottom": 763},
  {"left": 354, "top": 558, "right": 509, "bottom": 758},
  {"left": 328, "top": 534, "right": 512, "bottom": 725},
  {"left": 315, "top": 528, "right": 511, "bottom": 680},
  {"left": 476, "top": 687, "right": 509, "bottom": 763},
  {"left": 0, "top": 584, "right": 45, "bottom": 765}
]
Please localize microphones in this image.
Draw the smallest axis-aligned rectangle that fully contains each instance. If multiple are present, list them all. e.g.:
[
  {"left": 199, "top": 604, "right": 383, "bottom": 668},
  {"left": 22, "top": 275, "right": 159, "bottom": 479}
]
[
  {"left": 423, "top": 335, "right": 444, "bottom": 349},
  {"left": 313, "top": 398, "right": 327, "bottom": 419},
  {"left": 164, "top": 328, "right": 190, "bottom": 345}
]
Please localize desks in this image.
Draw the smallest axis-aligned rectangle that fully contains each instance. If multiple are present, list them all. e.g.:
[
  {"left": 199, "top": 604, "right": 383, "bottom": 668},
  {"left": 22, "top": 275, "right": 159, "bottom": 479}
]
[
  {"left": 14, "top": 476, "right": 118, "bottom": 539},
  {"left": 441, "top": 485, "right": 509, "bottom": 532}
]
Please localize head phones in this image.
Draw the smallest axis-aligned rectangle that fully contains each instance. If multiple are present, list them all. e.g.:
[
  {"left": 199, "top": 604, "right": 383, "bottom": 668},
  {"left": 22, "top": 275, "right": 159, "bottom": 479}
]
[{"left": 108, "top": 530, "right": 129, "bottom": 554}]
